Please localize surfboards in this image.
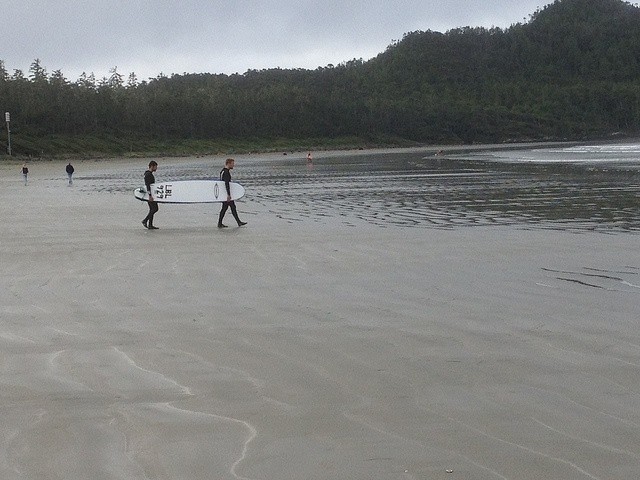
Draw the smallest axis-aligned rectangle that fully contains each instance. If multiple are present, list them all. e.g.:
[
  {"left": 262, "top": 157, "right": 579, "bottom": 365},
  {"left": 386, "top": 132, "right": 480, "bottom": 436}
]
[{"left": 133, "top": 180, "right": 245, "bottom": 204}]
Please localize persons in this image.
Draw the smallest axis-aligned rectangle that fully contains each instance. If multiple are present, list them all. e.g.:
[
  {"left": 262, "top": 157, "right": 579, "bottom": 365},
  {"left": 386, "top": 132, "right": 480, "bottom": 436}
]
[
  {"left": 142, "top": 160, "right": 159, "bottom": 230},
  {"left": 19, "top": 164, "right": 30, "bottom": 186},
  {"left": 217, "top": 158, "right": 248, "bottom": 228},
  {"left": 66, "top": 163, "right": 74, "bottom": 185}
]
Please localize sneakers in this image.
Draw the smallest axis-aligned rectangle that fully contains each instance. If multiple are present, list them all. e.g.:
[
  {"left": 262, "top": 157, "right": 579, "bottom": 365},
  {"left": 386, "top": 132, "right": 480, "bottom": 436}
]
[
  {"left": 148, "top": 226, "right": 160, "bottom": 230},
  {"left": 142, "top": 221, "right": 148, "bottom": 228},
  {"left": 238, "top": 222, "right": 248, "bottom": 227},
  {"left": 217, "top": 224, "right": 229, "bottom": 229}
]
